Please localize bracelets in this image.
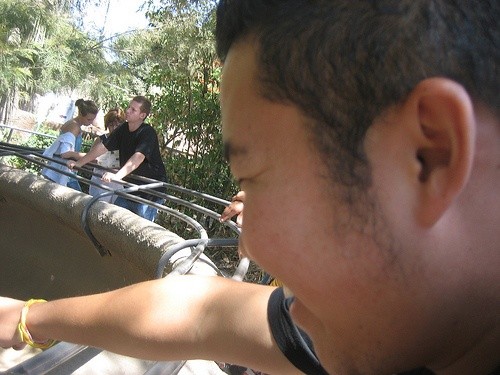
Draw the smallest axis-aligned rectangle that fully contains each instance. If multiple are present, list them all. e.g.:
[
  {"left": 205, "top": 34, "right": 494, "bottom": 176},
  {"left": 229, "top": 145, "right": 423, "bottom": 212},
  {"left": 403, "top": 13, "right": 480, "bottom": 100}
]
[{"left": 16, "top": 297, "right": 58, "bottom": 352}]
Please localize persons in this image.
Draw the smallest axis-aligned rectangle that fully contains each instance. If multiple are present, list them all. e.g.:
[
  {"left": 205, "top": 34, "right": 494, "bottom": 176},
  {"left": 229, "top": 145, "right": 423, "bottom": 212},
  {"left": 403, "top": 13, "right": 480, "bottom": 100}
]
[
  {"left": 43, "top": 98, "right": 98, "bottom": 193},
  {"left": 65, "top": 96, "right": 166, "bottom": 222},
  {"left": 218, "top": 190, "right": 287, "bottom": 287},
  {"left": 89, "top": 108, "right": 127, "bottom": 205},
  {"left": 1, "top": 1, "right": 500, "bottom": 375}
]
